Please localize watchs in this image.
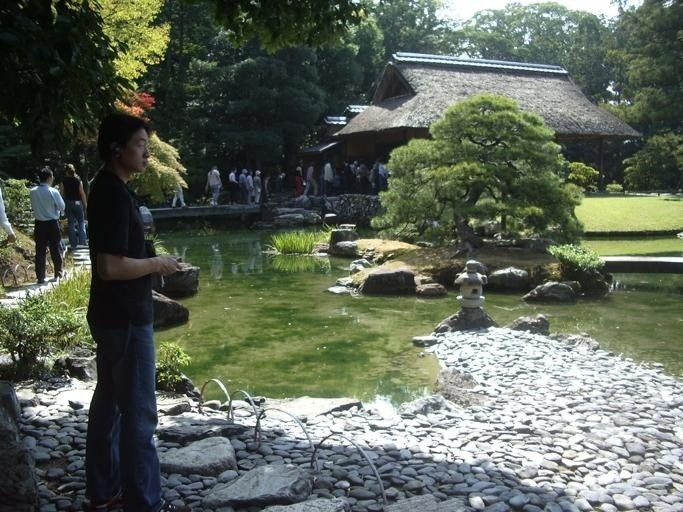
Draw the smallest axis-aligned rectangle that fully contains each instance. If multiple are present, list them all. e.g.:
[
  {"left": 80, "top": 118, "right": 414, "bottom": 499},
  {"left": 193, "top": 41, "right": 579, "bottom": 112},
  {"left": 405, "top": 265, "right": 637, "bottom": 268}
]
[{"left": 0, "top": 185, "right": 18, "bottom": 244}]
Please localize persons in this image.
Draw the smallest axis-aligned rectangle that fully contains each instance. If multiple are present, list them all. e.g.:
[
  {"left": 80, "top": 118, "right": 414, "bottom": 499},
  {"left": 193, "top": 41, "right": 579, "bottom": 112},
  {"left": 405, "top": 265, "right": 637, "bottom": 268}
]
[
  {"left": 29, "top": 168, "right": 66, "bottom": 285},
  {"left": 59, "top": 164, "right": 88, "bottom": 248},
  {"left": 203, "top": 164, "right": 224, "bottom": 206},
  {"left": 229, "top": 159, "right": 387, "bottom": 205},
  {"left": 82, "top": 110, "right": 190, "bottom": 512},
  {"left": 169, "top": 169, "right": 186, "bottom": 208}
]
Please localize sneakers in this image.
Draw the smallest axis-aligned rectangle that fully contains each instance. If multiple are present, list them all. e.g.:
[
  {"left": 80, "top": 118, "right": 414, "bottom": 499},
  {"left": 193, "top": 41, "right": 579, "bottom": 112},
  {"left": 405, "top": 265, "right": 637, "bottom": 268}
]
[
  {"left": 158, "top": 500, "right": 194, "bottom": 512},
  {"left": 89, "top": 484, "right": 128, "bottom": 512}
]
